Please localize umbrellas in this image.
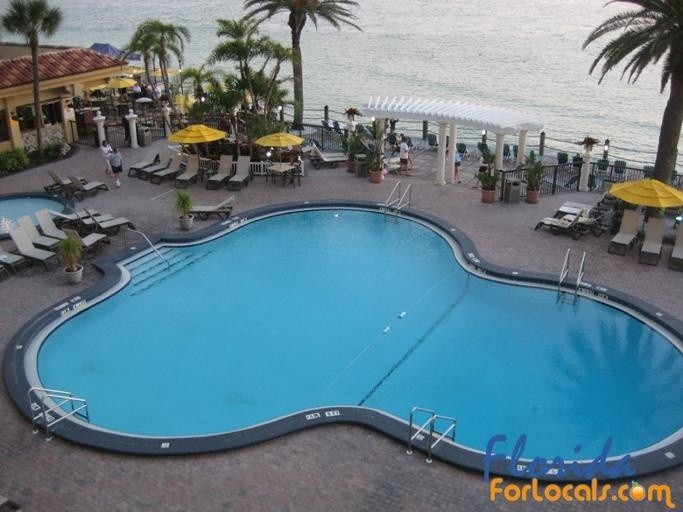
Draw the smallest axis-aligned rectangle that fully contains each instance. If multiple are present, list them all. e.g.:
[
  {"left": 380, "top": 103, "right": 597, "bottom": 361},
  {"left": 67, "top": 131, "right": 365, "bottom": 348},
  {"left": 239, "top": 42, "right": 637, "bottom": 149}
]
[
  {"left": 89, "top": 67, "right": 254, "bottom": 108},
  {"left": 167, "top": 123, "right": 226, "bottom": 154},
  {"left": 608, "top": 176, "right": 682, "bottom": 244},
  {"left": 252, "top": 133, "right": 305, "bottom": 166}
]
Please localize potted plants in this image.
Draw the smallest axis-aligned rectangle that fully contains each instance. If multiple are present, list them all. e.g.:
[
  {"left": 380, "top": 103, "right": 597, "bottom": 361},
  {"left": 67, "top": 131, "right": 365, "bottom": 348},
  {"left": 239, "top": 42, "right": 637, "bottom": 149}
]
[
  {"left": 520, "top": 150, "right": 543, "bottom": 204},
  {"left": 345, "top": 132, "right": 358, "bottom": 172},
  {"left": 175, "top": 191, "right": 196, "bottom": 230},
  {"left": 368, "top": 133, "right": 395, "bottom": 182},
  {"left": 476, "top": 168, "right": 501, "bottom": 204}
]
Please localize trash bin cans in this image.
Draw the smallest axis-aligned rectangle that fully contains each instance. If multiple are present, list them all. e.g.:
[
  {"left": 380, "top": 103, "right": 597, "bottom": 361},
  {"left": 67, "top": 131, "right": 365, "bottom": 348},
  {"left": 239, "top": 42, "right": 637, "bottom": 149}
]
[
  {"left": 504, "top": 177, "right": 520, "bottom": 203},
  {"left": 354, "top": 154, "right": 368, "bottom": 177},
  {"left": 138, "top": 126, "right": 153, "bottom": 146}
]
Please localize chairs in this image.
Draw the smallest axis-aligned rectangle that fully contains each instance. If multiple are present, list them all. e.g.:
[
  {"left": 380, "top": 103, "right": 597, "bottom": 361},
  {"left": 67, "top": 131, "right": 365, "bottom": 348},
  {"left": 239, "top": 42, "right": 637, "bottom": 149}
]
[
  {"left": 1, "top": 168, "right": 136, "bottom": 283},
  {"left": 533, "top": 187, "right": 683, "bottom": 272},
  {"left": 128, "top": 144, "right": 301, "bottom": 191},
  {"left": 76, "top": 77, "right": 189, "bottom": 129},
  {"left": 303, "top": 121, "right": 419, "bottom": 167},
  {"left": 427, "top": 134, "right": 677, "bottom": 188},
  {"left": 188, "top": 193, "right": 236, "bottom": 220}
]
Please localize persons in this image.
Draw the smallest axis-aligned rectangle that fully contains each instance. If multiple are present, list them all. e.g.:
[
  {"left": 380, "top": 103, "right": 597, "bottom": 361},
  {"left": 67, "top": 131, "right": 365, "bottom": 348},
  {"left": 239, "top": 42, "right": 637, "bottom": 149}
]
[
  {"left": 446, "top": 144, "right": 461, "bottom": 185},
  {"left": 397, "top": 137, "right": 412, "bottom": 176},
  {"left": 471, "top": 153, "right": 489, "bottom": 188},
  {"left": 100, "top": 140, "right": 115, "bottom": 178},
  {"left": 109, "top": 148, "right": 124, "bottom": 189}
]
